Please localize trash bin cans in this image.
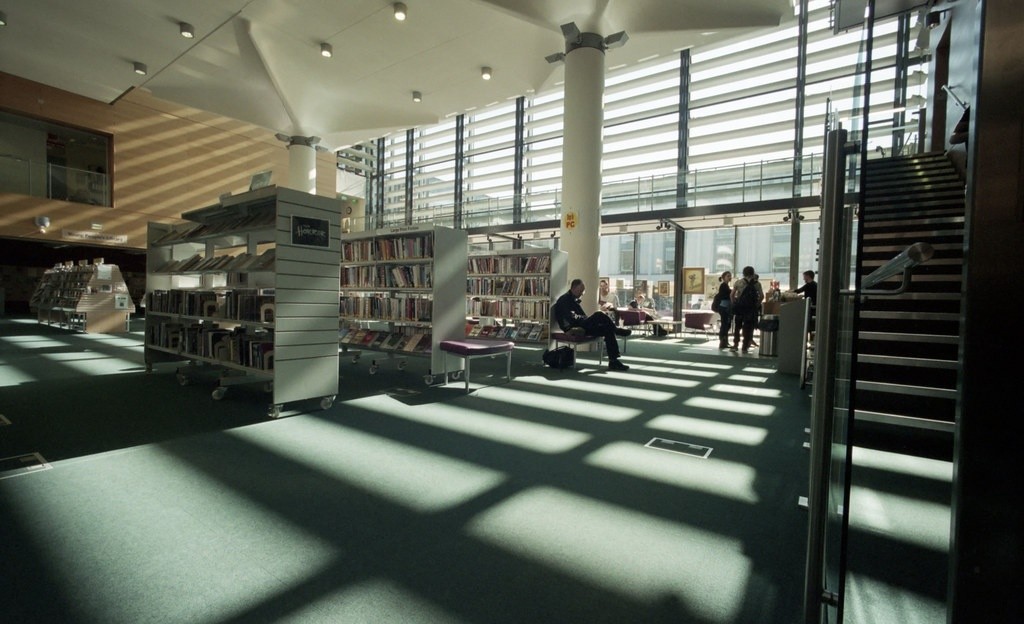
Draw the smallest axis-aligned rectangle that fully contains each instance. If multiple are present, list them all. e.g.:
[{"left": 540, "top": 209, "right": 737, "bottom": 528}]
[{"left": 759, "top": 314, "right": 779, "bottom": 357}]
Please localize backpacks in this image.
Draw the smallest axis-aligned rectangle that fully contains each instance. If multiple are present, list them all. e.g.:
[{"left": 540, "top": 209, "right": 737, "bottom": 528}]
[
  {"left": 543, "top": 345, "right": 574, "bottom": 371},
  {"left": 739, "top": 278, "right": 758, "bottom": 313}
]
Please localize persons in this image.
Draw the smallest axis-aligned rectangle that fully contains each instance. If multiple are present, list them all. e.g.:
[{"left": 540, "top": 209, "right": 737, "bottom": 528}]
[
  {"left": 719, "top": 271, "right": 735, "bottom": 349},
  {"left": 599, "top": 280, "right": 622, "bottom": 323},
  {"left": 628, "top": 293, "right": 656, "bottom": 322},
  {"left": 791, "top": 270, "right": 818, "bottom": 357},
  {"left": 555, "top": 279, "right": 632, "bottom": 371},
  {"left": 730, "top": 266, "right": 765, "bottom": 354},
  {"left": 768, "top": 281, "right": 783, "bottom": 301},
  {"left": 688, "top": 274, "right": 696, "bottom": 290}
]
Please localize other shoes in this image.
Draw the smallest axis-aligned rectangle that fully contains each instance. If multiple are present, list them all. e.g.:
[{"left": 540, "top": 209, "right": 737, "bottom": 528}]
[
  {"left": 609, "top": 360, "right": 629, "bottom": 370},
  {"left": 742, "top": 351, "right": 748, "bottom": 353},
  {"left": 807, "top": 341, "right": 811, "bottom": 348},
  {"left": 731, "top": 346, "right": 738, "bottom": 351},
  {"left": 720, "top": 343, "right": 732, "bottom": 347},
  {"left": 615, "top": 329, "right": 631, "bottom": 336},
  {"left": 751, "top": 340, "right": 760, "bottom": 347}
]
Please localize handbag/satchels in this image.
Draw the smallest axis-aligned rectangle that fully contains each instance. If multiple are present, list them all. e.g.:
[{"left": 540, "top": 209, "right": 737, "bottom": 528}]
[{"left": 711, "top": 293, "right": 721, "bottom": 312}]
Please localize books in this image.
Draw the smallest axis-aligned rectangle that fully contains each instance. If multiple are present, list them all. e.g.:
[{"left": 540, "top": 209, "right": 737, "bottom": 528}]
[
  {"left": 152, "top": 286, "right": 275, "bottom": 370},
  {"left": 466, "top": 256, "right": 549, "bottom": 341},
  {"left": 150, "top": 203, "right": 275, "bottom": 243},
  {"left": 153, "top": 248, "right": 275, "bottom": 270},
  {"left": 121, "top": 274, "right": 146, "bottom": 305},
  {"left": 339, "top": 235, "right": 432, "bottom": 353},
  {"left": 31, "top": 264, "right": 95, "bottom": 308}
]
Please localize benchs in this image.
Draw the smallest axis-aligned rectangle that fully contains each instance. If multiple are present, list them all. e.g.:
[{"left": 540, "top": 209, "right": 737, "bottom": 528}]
[
  {"left": 554, "top": 334, "right": 606, "bottom": 368},
  {"left": 440, "top": 339, "right": 513, "bottom": 395}
]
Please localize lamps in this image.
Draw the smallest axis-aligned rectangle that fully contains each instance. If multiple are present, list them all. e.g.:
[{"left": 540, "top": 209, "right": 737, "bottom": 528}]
[
  {"left": 552, "top": 231, "right": 556, "bottom": 238},
  {"left": 412, "top": 92, "right": 421, "bottom": 102},
  {"left": 275, "top": 133, "right": 323, "bottom": 151},
  {"left": 181, "top": 23, "right": 195, "bottom": 38},
  {"left": 0, "top": 12, "right": 7, "bottom": 26},
  {"left": 482, "top": 67, "right": 491, "bottom": 80},
  {"left": 545, "top": 23, "right": 629, "bottom": 66},
  {"left": 393, "top": 1, "right": 407, "bottom": 21},
  {"left": 656, "top": 220, "right": 670, "bottom": 230},
  {"left": 321, "top": 43, "right": 332, "bottom": 57},
  {"left": 925, "top": 5, "right": 954, "bottom": 28},
  {"left": 133, "top": 62, "right": 146, "bottom": 75},
  {"left": 486, "top": 235, "right": 492, "bottom": 242},
  {"left": 783, "top": 209, "right": 803, "bottom": 223},
  {"left": 517, "top": 234, "right": 522, "bottom": 240}
]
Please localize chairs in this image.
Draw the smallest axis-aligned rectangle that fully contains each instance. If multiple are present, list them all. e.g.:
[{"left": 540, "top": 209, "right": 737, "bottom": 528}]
[{"left": 614, "top": 308, "right": 715, "bottom": 341}]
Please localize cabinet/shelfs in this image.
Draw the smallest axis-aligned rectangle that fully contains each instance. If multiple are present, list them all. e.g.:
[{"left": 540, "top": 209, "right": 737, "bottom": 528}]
[{"left": 28, "top": 184, "right": 568, "bottom": 418}]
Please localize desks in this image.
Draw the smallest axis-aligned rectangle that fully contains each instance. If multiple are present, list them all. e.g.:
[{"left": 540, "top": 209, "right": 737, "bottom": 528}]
[{"left": 647, "top": 320, "right": 682, "bottom": 339}]
